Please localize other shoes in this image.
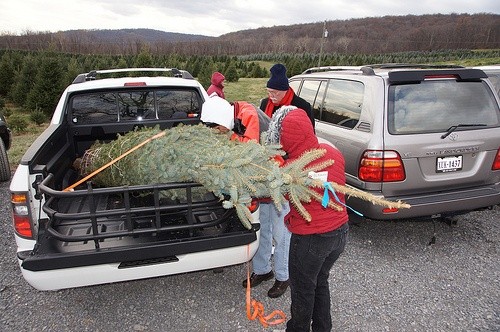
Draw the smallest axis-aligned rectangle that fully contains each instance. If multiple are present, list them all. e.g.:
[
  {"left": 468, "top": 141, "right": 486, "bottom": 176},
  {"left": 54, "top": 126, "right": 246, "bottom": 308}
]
[
  {"left": 242, "top": 270, "right": 274, "bottom": 288},
  {"left": 268, "top": 279, "right": 290, "bottom": 298}
]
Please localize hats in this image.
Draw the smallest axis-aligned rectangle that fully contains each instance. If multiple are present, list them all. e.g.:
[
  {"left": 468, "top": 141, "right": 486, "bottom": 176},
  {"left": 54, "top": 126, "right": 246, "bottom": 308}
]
[
  {"left": 200, "top": 95, "right": 234, "bottom": 131},
  {"left": 266, "top": 64, "right": 289, "bottom": 91}
]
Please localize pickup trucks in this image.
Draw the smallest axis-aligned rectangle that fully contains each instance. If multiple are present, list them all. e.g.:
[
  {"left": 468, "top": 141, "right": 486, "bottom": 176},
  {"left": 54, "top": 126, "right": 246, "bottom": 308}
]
[{"left": 10, "top": 66, "right": 262, "bottom": 293}]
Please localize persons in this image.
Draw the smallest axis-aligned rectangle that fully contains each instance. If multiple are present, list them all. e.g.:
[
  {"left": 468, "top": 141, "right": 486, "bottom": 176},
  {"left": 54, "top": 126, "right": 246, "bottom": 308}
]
[
  {"left": 201, "top": 95, "right": 292, "bottom": 297},
  {"left": 266, "top": 105, "right": 349, "bottom": 332},
  {"left": 260, "top": 63, "right": 314, "bottom": 159},
  {"left": 207, "top": 72, "right": 225, "bottom": 99}
]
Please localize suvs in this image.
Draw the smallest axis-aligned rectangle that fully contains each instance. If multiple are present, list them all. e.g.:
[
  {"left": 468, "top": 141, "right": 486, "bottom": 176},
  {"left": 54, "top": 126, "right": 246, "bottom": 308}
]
[{"left": 280, "top": 63, "right": 500, "bottom": 225}]
